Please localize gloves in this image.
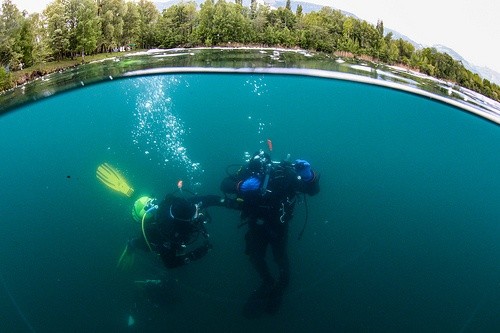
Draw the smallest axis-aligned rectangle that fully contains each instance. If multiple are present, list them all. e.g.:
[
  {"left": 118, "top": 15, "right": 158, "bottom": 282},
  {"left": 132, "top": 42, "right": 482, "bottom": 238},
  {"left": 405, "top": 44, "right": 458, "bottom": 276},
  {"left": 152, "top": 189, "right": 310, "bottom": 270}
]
[
  {"left": 240, "top": 177, "right": 261, "bottom": 192},
  {"left": 294, "top": 159, "right": 313, "bottom": 181}
]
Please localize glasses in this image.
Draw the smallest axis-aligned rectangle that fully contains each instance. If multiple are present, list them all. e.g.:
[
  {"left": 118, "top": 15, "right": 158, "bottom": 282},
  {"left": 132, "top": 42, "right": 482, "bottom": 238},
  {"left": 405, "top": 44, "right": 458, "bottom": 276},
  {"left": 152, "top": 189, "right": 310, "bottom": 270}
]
[{"left": 192, "top": 203, "right": 199, "bottom": 221}]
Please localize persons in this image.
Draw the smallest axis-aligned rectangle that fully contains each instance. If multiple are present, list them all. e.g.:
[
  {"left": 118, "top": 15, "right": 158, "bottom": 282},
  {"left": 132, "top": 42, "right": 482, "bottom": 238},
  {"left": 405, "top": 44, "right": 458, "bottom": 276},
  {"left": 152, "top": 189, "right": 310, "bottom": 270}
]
[
  {"left": 126, "top": 45, "right": 131, "bottom": 52},
  {"left": 113, "top": 46, "right": 118, "bottom": 52},
  {"left": 221, "top": 153, "right": 321, "bottom": 319},
  {"left": 120, "top": 44, "right": 125, "bottom": 52},
  {"left": 129, "top": 194, "right": 245, "bottom": 279}
]
